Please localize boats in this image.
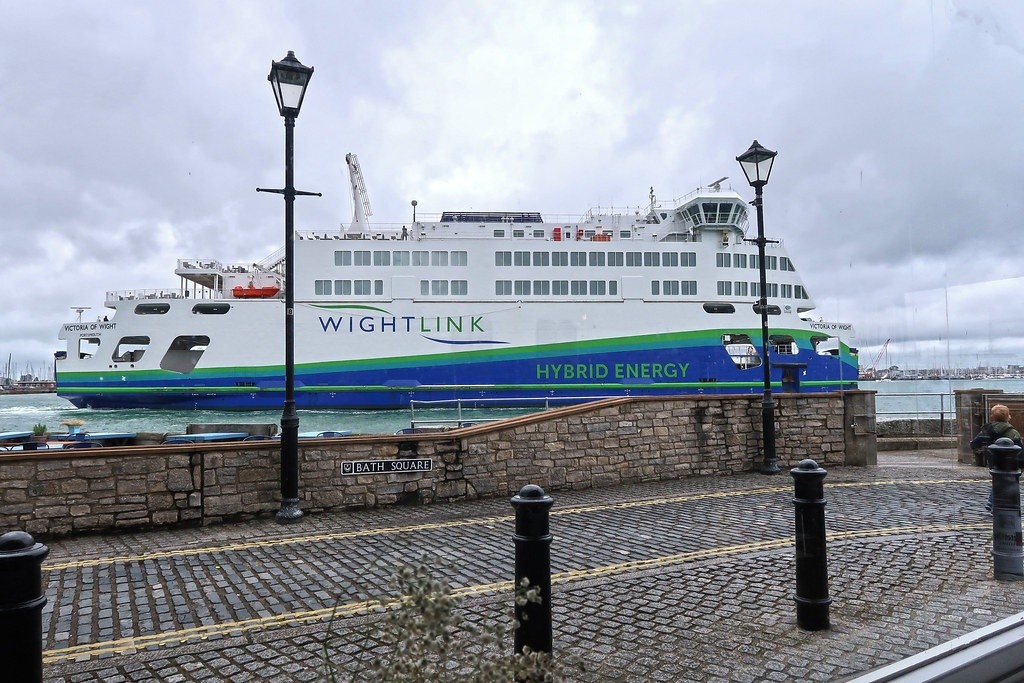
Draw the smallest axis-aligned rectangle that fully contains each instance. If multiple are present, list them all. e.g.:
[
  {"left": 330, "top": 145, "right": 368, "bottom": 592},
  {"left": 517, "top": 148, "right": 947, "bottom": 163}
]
[{"left": 231, "top": 275, "right": 281, "bottom": 299}]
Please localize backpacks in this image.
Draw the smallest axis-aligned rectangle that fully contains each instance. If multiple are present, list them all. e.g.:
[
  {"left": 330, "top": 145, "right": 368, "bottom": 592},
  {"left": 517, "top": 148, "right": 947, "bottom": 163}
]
[{"left": 987, "top": 423, "right": 1015, "bottom": 444}]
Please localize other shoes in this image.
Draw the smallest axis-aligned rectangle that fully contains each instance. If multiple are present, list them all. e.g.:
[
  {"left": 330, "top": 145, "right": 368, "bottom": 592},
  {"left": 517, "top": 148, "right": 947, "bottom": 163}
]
[{"left": 985, "top": 504, "right": 992, "bottom": 511}]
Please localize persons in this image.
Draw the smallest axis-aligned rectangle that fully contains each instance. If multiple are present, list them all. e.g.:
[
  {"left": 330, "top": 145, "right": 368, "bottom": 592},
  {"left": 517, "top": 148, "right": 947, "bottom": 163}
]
[
  {"left": 103, "top": 316, "right": 109, "bottom": 321},
  {"left": 972, "top": 404, "right": 1024, "bottom": 514},
  {"left": 402, "top": 226, "right": 408, "bottom": 241}
]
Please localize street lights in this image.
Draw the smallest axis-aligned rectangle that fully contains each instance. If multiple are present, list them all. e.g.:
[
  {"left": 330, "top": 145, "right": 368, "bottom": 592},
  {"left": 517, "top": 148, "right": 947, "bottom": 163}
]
[
  {"left": 249, "top": 50, "right": 324, "bottom": 525},
  {"left": 735, "top": 138, "right": 794, "bottom": 479}
]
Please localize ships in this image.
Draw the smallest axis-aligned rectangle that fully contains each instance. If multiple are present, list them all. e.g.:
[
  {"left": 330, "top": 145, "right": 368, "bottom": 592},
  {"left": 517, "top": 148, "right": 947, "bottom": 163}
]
[{"left": 52, "top": 153, "right": 861, "bottom": 416}]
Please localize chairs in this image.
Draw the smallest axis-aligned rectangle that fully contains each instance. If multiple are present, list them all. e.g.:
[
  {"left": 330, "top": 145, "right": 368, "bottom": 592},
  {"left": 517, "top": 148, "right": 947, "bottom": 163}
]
[
  {"left": 65, "top": 442, "right": 104, "bottom": 449},
  {"left": 395, "top": 428, "right": 423, "bottom": 434},
  {"left": 65, "top": 432, "right": 91, "bottom": 442},
  {"left": 316, "top": 431, "right": 344, "bottom": 438},
  {"left": 243, "top": 435, "right": 274, "bottom": 441},
  {"left": 10, "top": 442, "right": 49, "bottom": 450}
]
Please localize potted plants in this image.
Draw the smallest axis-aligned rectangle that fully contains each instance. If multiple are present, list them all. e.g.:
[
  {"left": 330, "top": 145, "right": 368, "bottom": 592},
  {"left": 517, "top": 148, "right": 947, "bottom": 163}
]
[{"left": 30, "top": 424, "right": 48, "bottom": 446}]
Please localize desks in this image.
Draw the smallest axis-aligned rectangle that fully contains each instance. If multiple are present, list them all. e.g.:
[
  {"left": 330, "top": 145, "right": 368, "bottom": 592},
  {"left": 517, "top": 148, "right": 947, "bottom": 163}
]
[
  {"left": 167, "top": 433, "right": 250, "bottom": 442},
  {"left": 0, "top": 440, "right": 101, "bottom": 452},
  {"left": 271, "top": 430, "right": 352, "bottom": 440}
]
